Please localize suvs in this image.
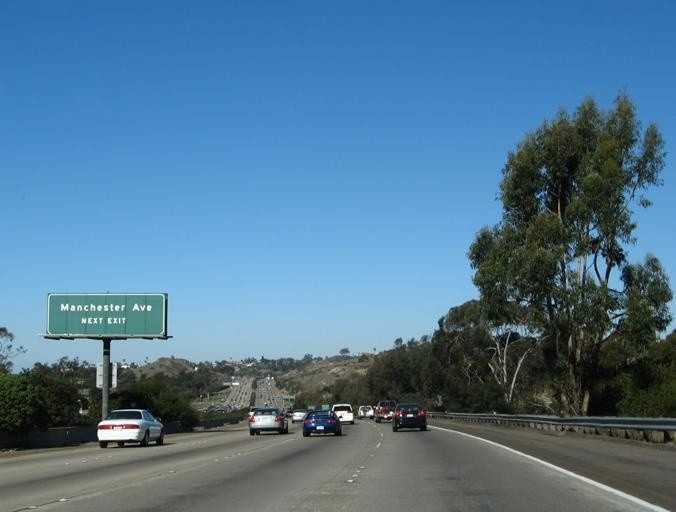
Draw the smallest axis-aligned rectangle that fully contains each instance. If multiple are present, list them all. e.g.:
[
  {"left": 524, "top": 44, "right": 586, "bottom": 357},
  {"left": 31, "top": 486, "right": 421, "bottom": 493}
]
[{"left": 391, "top": 403, "right": 429, "bottom": 434}]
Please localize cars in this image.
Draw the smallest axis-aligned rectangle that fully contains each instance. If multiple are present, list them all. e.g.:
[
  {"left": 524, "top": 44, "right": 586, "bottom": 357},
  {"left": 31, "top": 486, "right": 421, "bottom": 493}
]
[
  {"left": 247, "top": 409, "right": 289, "bottom": 436},
  {"left": 95, "top": 408, "right": 164, "bottom": 449},
  {"left": 247, "top": 400, "right": 397, "bottom": 427},
  {"left": 198, "top": 375, "right": 283, "bottom": 414},
  {"left": 302, "top": 409, "right": 343, "bottom": 438}
]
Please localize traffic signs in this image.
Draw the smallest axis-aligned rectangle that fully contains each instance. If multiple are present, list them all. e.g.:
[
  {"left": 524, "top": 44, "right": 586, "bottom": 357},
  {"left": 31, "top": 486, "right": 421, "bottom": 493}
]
[{"left": 45, "top": 291, "right": 167, "bottom": 338}]
